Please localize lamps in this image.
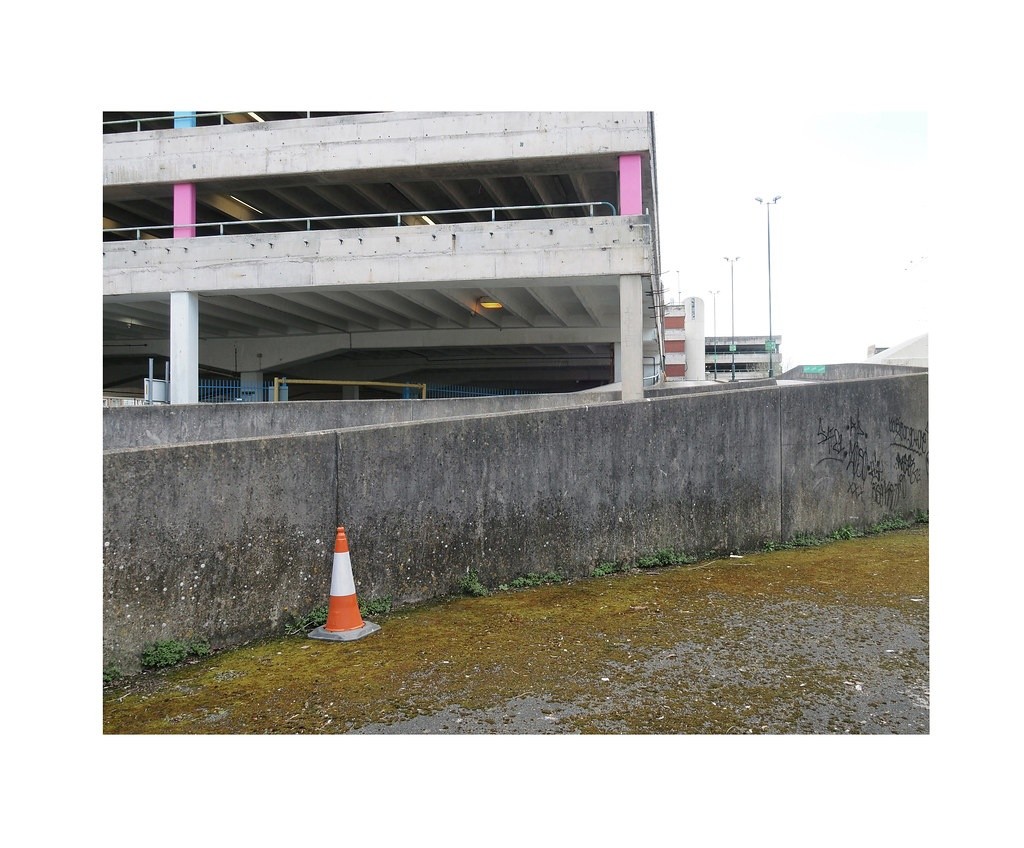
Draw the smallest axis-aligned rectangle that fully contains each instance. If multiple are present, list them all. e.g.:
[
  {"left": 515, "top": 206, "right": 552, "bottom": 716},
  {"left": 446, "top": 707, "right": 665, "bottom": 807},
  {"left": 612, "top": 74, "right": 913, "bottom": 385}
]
[{"left": 480, "top": 296, "right": 502, "bottom": 309}]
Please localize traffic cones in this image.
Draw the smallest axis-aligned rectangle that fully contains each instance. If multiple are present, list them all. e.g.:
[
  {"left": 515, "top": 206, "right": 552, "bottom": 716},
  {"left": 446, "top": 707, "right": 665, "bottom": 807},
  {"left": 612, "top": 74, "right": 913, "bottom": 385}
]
[{"left": 308, "top": 526, "right": 382, "bottom": 641}]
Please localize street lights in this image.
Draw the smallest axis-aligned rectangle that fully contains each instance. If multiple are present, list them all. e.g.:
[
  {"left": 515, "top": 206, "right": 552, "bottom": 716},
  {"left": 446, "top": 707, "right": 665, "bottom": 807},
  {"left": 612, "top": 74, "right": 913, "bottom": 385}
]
[
  {"left": 755, "top": 195, "right": 782, "bottom": 378},
  {"left": 724, "top": 256, "right": 741, "bottom": 381}
]
